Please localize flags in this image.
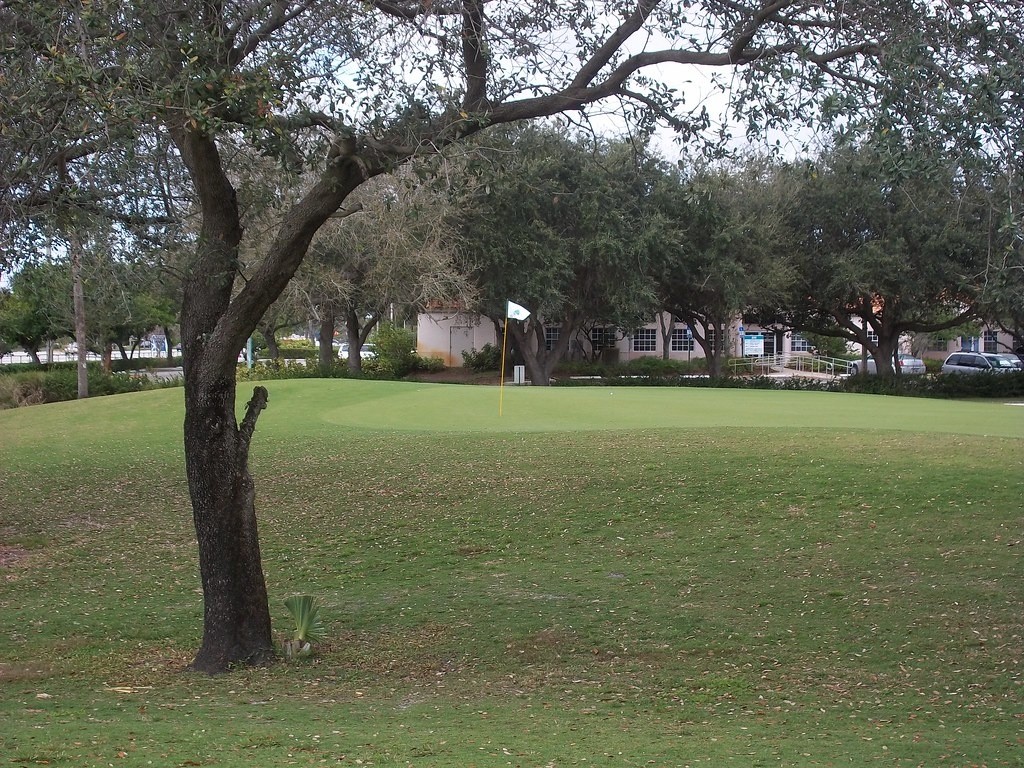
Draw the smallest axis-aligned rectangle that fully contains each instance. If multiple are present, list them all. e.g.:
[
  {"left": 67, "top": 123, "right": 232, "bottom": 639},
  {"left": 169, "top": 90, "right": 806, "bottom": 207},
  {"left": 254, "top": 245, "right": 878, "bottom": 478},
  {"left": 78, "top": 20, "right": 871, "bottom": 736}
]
[{"left": 508, "top": 300, "right": 531, "bottom": 321}]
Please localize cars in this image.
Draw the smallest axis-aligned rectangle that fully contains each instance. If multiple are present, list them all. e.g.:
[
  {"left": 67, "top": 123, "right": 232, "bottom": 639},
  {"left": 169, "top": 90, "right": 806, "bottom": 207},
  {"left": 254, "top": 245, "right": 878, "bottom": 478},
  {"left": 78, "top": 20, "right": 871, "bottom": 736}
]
[
  {"left": 997, "top": 353, "right": 1023, "bottom": 368},
  {"left": 848, "top": 354, "right": 926, "bottom": 377},
  {"left": 176, "top": 343, "right": 182, "bottom": 351},
  {"left": 337, "top": 342, "right": 378, "bottom": 361},
  {"left": 64, "top": 342, "right": 78, "bottom": 356},
  {"left": 140, "top": 341, "right": 151, "bottom": 348}
]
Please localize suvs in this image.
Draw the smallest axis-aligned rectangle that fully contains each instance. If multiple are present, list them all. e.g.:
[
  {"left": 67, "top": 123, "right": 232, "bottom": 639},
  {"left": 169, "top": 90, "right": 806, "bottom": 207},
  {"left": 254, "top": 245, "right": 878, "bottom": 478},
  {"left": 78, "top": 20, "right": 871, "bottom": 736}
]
[{"left": 941, "top": 350, "right": 1022, "bottom": 375}]
